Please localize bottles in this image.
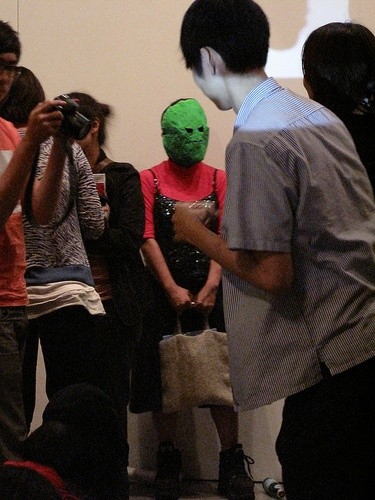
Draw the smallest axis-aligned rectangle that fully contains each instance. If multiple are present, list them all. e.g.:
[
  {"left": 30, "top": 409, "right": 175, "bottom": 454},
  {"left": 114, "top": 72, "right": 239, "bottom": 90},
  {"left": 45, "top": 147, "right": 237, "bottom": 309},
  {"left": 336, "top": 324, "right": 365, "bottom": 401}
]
[{"left": 262, "top": 477, "right": 285, "bottom": 499}]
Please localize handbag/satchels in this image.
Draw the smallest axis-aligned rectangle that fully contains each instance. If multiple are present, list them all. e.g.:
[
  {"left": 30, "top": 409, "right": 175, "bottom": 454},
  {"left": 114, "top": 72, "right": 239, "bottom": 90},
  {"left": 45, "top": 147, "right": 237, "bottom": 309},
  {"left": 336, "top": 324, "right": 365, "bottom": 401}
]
[{"left": 161, "top": 301, "right": 238, "bottom": 408}]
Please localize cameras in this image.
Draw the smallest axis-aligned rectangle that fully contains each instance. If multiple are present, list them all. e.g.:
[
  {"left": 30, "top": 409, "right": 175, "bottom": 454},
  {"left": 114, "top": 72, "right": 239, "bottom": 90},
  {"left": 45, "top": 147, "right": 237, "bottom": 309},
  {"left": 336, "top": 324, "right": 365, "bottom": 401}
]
[{"left": 48, "top": 94, "right": 91, "bottom": 141}]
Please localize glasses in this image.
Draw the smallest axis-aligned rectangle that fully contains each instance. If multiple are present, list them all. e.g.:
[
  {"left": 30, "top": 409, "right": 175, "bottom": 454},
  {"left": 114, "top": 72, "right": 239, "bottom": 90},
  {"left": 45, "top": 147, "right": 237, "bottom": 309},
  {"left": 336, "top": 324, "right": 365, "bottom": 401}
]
[{"left": 1, "top": 64, "right": 23, "bottom": 78}]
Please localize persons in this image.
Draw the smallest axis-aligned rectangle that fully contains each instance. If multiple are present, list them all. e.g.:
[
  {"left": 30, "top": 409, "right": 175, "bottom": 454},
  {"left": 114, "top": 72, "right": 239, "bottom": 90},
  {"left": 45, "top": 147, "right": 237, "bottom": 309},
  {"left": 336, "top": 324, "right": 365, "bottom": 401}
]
[
  {"left": 50, "top": 92, "right": 145, "bottom": 500},
  {"left": 301, "top": 22, "right": 375, "bottom": 199},
  {"left": 0, "top": 66, "right": 130, "bottom": 468},
  {"left": 0, "top": 457, "right": 78, "bottom": 499},
  {"left": 138, "top": 98, "right": 256, "bottom": 499},
  {"left": 25, "top": 384, "right": 133, "bottom": 500},
  {"left": 0, "top": 21, "right": 80, "bottom": 467},
  {"left": 172, "top": 1, "right": 375, "bottom": 500}
]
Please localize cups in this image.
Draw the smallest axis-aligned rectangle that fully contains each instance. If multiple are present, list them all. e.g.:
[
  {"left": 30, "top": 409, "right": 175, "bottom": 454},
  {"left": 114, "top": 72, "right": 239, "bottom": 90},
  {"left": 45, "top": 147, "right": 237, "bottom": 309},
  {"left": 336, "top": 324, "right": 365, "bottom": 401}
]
[
  {"left": 93, "top": 173, "right": 106, "bottom": 206},
  {"left": 175, "top": 201, "right": 215, "bottom": 254}
]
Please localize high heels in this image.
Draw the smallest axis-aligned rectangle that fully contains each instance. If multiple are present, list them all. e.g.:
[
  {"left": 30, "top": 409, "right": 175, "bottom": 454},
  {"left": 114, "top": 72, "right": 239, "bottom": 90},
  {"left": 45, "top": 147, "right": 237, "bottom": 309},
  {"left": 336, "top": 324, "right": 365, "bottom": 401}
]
[
  {"left": 216, "top": 439, "right": 256, "bottom": 499},
  {"left": 156, "top": 451, "right": 191, "bottom": 499}
]
